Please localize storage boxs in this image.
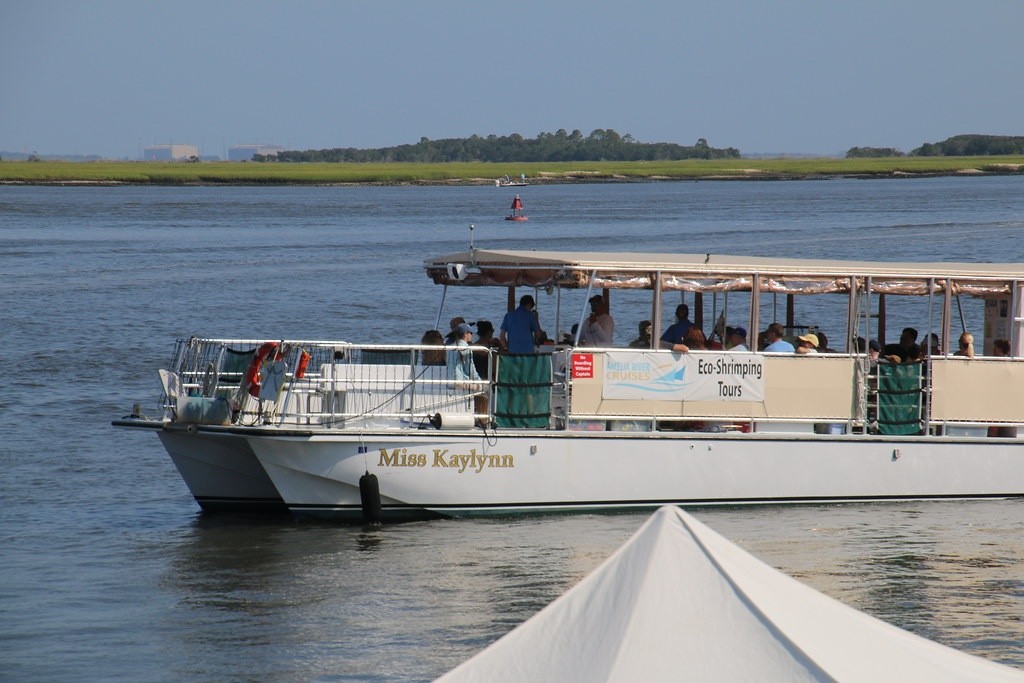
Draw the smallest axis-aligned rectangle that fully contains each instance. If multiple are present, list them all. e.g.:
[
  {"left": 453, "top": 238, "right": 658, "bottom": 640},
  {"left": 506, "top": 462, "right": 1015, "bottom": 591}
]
[
  {"left": 704, "top": 420, "right": 734, "bottom": 432},
  {"left": 555, "top": 402, "right": 653, "bottom": 431},
  {"left": 823, "top": 423, "right": 845, "bottom": 435}
]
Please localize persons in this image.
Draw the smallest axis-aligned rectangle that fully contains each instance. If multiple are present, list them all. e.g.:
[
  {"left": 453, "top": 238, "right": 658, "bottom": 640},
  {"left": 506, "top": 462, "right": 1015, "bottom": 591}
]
[
  {"left": 500, "top": 295, "right": 543, "bottom": 354},
  {"left": 630, "top": 304, "right": 749, "bottom": 353},
  {"left": 859, "top": 327, "right": 940, "bottom": 362},
  {"left": 758, "top": 323, "right": 836, "bottom": 353},
  {"left": 953, "top": 333, "right": 974, "bottom": 358},
  {"left": 993, "top": 339, "right": 1011, "bottom": 357},
  {"left": 423, "top": 317, "right": 500, "bottom": 428},
  {"left": 564, "top": 295, "right": 614, "bottom": 348}
]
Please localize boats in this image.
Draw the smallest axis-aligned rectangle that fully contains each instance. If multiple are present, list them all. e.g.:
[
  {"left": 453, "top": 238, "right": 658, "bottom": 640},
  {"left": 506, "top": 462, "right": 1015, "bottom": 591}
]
[
  {"left": 111, "top": 247, "right": 1024, "bottom": 520},
  {"left": 500, "top": 181, "right": 530, "bottom": 186}
]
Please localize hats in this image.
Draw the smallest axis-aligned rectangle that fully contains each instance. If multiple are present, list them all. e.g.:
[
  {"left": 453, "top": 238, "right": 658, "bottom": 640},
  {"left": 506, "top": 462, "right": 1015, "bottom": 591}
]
[
  {"left": 589, "top": 295, "right": 604, "bottom": 304},
  {"left": 457, "top": 323, "right": 478, "bottom": 336},
  {"left": 445, "top": 317, "right": 476, "bottom": 338},
  {"left": 799, "top": 334, "right": 819, "bottom": 347},
  {"left": 521, "top": 295, "right": 535, "bottom": 305},
  {"left": 729, "top": 327, "right": 747, "bottom": 338}
]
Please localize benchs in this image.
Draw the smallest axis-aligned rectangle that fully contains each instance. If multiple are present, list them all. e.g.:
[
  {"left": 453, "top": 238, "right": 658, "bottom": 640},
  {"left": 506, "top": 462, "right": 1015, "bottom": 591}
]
[{"left": 230, "top": 361, "right": 475, "bottom": 426}]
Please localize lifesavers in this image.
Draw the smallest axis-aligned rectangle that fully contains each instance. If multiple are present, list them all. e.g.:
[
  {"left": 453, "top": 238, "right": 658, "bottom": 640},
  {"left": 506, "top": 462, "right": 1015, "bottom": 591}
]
[{"left": 246, "top": 342, "right": 281, "bottom": 398}]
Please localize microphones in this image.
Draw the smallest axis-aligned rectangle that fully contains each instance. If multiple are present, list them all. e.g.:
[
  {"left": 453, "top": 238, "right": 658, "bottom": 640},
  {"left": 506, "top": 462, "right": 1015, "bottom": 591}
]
[{"left": 589, "top": 312, "right": 596, "bottom": 327}]
